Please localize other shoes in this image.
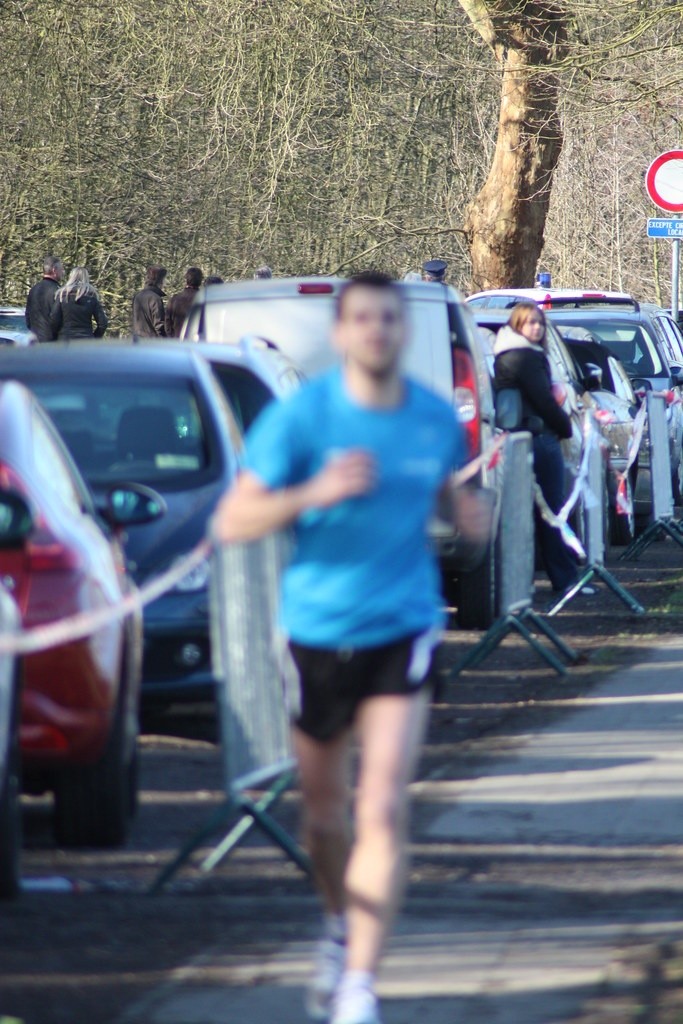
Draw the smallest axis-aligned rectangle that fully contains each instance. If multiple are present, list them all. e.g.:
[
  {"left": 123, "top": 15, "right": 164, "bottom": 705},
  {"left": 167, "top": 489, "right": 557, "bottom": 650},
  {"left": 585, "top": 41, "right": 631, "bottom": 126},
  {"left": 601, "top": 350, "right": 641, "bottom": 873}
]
[
  {"left": 551, "top": 577, "right": 604, "bottom": 599},
  {"left": 303, "top": 936, "right": 345, "bottom": 1019},
  {"left": 328, "top": 980, "right": 380, "bottom": 1024}
]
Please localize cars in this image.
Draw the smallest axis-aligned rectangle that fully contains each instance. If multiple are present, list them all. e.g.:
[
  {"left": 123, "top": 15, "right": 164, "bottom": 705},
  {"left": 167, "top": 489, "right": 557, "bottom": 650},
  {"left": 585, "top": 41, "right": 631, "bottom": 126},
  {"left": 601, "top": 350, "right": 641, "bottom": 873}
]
[
  {"left": 2, "top": 260, "right": 683, "bottom": 703},
  {"left": 0, "top": 380, "right": 170, "bottom": 847}
]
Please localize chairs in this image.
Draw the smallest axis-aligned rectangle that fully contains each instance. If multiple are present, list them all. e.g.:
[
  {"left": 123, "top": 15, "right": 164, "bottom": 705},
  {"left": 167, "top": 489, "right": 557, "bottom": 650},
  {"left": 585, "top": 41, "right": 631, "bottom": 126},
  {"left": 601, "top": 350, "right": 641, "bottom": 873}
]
[
  {"left": 117, "top": 405, "right": 180, "bottom": 462},
  {"left": 600, "top": 339, "right": 642, "bottom": 372}
]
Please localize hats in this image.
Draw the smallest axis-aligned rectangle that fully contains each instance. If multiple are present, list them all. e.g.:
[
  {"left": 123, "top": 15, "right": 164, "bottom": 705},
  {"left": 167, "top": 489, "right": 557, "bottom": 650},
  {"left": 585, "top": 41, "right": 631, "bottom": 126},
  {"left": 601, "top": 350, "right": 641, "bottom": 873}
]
[{"left": 423, "top": 260, "right": 447, "bottom": 277}]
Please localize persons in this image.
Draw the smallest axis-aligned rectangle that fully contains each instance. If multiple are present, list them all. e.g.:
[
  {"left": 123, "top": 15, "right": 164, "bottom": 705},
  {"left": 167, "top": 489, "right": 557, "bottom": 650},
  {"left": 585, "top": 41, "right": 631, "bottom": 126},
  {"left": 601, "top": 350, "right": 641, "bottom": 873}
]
[
  {"left": 25, "top": 256, "right": 107, "bottom": 343},
  {"left": 492, "top": 301, "right": 602, "bottom": 595},
  {"left": 253, "top": 266, "right": 272, "bottom": 280},
  {"left": 204, "top": 272, "right": 492, "bottom": 1024},
  {"left": 423, "top": 260, "right": 447, "bottom": 282},
  {"left": 133, "top": 265, "right": 224, "bottom": 340}
]
